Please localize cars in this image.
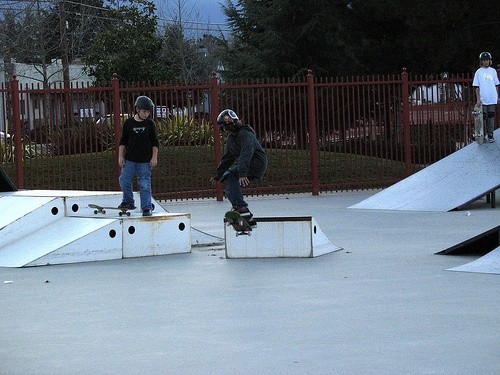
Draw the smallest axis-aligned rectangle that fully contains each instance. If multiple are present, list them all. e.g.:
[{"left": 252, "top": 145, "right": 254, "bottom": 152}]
[{"left": 95, "top": 113, "right": 131, "bottom": 126}]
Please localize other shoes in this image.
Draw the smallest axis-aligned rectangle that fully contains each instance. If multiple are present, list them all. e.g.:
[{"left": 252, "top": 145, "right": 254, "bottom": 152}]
[
  {"left": 483, "top": 138, "right": 495, "bottom": 143},
  {"left": 142, "top": 209, "right": 152, "bottom": 216},
  {"left": 118, "top": 202, "right": 136, "bottom": 208}
]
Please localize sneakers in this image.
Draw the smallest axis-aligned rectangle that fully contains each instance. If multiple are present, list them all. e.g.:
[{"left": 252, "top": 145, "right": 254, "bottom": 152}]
[
  {"left": 248, "top": 220, "right": 258, "bottom": 228},
  {"left": 224, "top": 207, "right": 250, "bottom": 218}
]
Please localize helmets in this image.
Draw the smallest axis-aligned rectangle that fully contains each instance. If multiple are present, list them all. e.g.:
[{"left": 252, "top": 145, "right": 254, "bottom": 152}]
[
  {"left": 216, "top": 108, "right": 239, "bottom": 129},
  {"left": 134, "top": 95, "right": 154, "bottom": 111},
  {"left": 479, "top": 51, "right": 492, "bottom": 60}
]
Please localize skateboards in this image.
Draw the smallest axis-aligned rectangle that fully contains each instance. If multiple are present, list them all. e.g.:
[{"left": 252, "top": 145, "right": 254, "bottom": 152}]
[
  {"left": 223, "top": 212, "right": 253, "bottom": 239},
  {"left": 473, "top": 104, "right": 485, "bottom": 145},
  {"left": 88, "top": 203, "right": 136, "bottom": 216}
]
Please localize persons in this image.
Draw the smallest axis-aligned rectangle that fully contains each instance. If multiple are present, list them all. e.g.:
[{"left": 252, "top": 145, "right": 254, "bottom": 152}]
[
  {"left": 118, "top": 96, "right": 160, "bottom": 216},
  {"left": 208, "top": 109, "right": 268, "bottom": 228},
  {"left": 472, "top": 52, "right": 500, "bottom": 143}
]
